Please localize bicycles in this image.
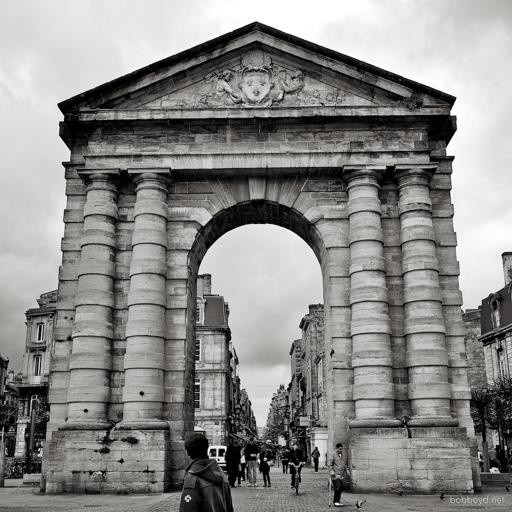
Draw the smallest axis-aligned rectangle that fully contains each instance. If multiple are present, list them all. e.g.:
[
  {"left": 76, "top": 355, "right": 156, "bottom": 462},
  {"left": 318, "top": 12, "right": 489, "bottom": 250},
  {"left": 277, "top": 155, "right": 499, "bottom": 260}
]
[
  {"left": 288, "top": 462, "right": 303, "bottom": 495},
  {"left": 4, "top": 460, "right": 23, "bottom": 477}
]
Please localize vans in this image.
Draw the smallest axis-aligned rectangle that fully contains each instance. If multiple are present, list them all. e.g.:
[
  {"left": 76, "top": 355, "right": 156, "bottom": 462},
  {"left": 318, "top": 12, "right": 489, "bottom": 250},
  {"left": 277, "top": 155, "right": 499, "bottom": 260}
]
[{"left": 208, "top": 446, "right": 228, "bottom": 468}]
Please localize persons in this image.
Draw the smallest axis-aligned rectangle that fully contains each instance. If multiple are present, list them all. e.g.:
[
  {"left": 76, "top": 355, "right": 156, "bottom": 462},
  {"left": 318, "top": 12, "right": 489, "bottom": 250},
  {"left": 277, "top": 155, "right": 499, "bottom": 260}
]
[
  {"left": 327, "top": 442, "right": 351, "bottom": 507},
  {"left": 178, "top": 432, "right": 235, "bottom": 511},
  {"left": 477, "top": 448, "right": 484, "bottom": 470},
  {"left": 198, "top": 62, "right": 307, "bottom": 109},
  {"left": 280, "top": 445, "right": 289, "bottom": 473},
  {"left": 223, "top": 441, "right": 239, "bottom": 488},
  {"left": 287, "top": 451, "right": 305, "bottom": 488},
  {"left": 234, "top": 438, "right": 275, "bottom": 487},
  {"left": 310, "top": 446, "right": 320, "bottom": 472}
]
